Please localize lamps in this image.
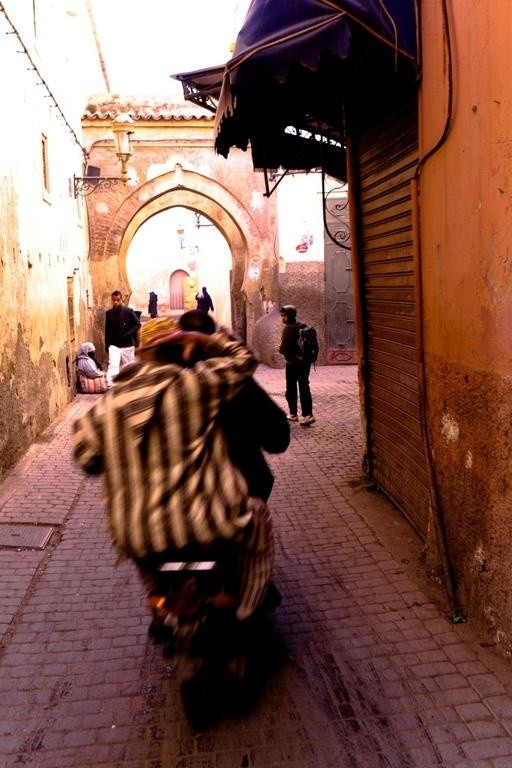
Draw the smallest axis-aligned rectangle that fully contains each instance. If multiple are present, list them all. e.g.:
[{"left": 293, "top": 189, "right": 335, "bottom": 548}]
[{"left": 74, "top": 111, "right": 137, "bottom": 199}]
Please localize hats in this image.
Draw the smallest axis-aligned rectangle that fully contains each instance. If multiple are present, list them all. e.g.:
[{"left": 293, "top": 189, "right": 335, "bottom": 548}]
[{"left": 133, "top": 315, "right": 184, "bottom": 354}]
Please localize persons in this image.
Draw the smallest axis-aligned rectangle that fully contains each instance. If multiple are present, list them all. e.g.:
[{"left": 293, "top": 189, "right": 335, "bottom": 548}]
[
  {"left": 275, "top": 304, "right": 315, "bottom": 427},
  {"left": 104, "top": 290, "right": 141, "bottom": 389},
  {"left": 70, "top": 313, "right": 276, "bottom": 627},
  {"left": 176, "top": 311, "right": 291, "bottom": 503},
  {"left": 195, "top": 286, "right": 214, "bottom": 315},
  {"left": 148, "top": 292, "right": 159, "bottom": 319},
  {"left": 77, "top": 342, "right": 109, "bottom": 393}
]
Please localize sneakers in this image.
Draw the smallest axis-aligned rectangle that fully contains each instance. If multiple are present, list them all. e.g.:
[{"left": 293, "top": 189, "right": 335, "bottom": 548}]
[{"left": 286, "top": 413, "right": 315, "bottom": 426}]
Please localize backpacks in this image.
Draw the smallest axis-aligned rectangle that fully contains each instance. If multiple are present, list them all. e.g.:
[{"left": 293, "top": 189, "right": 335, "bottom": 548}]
[{"left": 292, "top": 323, "right": 320, "bottom": 364}]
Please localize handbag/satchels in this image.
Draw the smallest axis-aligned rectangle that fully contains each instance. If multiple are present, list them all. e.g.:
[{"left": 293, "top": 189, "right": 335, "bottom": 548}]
[
  {"left": 78, "top": 370, "right": 108, "bottom": 394},
  {"left": 196, "top": 292, "right": 204, "bottom": 299}
]
